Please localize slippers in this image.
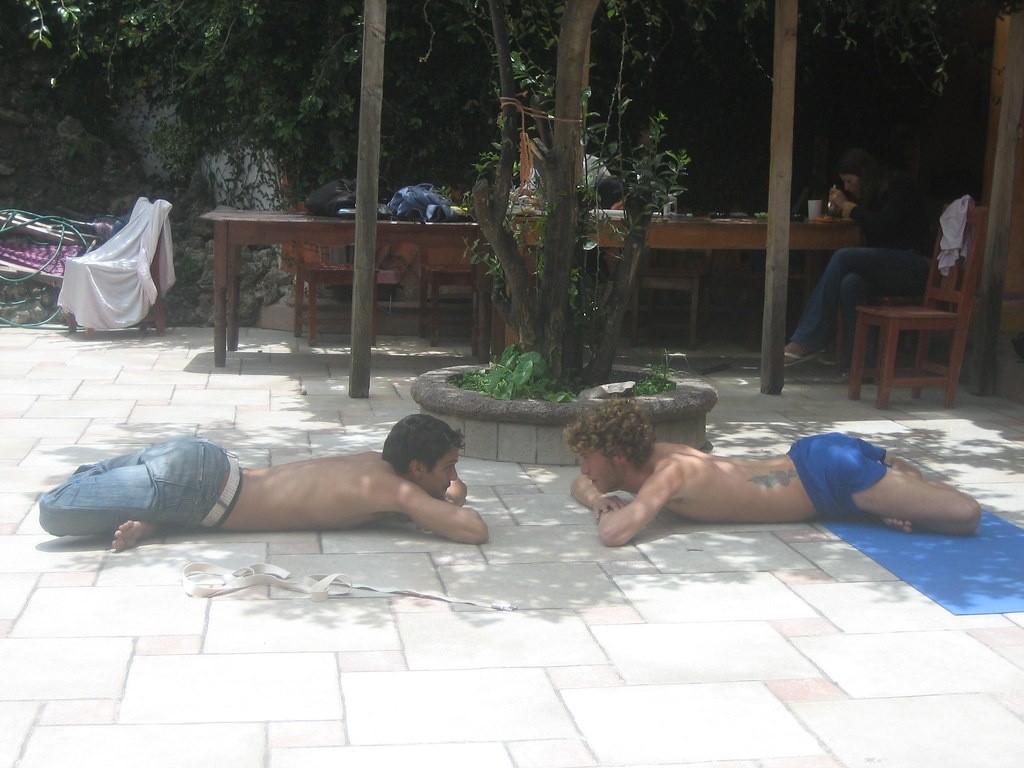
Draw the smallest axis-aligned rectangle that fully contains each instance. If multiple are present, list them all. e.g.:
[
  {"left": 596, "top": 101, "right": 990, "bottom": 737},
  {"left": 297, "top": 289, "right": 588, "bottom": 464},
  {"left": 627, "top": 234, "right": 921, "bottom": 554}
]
[
  {"left": 818, "top": 351, "right": 840, "bottom": 366},
  {"left": 785, "top": 345, "right": 824, "bottom": 367}
]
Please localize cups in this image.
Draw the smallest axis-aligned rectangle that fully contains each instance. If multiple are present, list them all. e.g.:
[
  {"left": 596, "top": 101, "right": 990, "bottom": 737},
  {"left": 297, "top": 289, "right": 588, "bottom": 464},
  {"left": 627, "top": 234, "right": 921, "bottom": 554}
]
[
  {"left": 808, "top": 200, "right": 822, "bottom": 220},
  {"left": 663, "top": 194, "right": 677, "bottom": 214}
]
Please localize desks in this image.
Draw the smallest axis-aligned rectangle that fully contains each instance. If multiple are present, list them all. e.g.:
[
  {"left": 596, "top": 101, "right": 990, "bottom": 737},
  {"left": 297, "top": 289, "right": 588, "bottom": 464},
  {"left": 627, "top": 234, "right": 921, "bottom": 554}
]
[
  {"left": 201, "top": 211, "right": 492, "bottom": 367},
  {"left": 503, "top": 216, "right": 861, "bottom": 367}
]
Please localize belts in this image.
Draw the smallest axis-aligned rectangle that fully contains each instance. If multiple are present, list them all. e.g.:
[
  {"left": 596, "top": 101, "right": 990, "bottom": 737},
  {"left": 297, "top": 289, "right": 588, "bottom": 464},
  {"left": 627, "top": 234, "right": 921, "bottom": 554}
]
[{"left": 199, "top": 450, "right": 240, "bottom": 530}]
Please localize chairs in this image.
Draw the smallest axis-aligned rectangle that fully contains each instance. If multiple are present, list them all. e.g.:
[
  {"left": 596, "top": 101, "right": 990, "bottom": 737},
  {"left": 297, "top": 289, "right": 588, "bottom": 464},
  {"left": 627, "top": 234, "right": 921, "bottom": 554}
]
[
  {"left": 629, "top": 208, "right": 804, "bottom": 350},
  {"left": 848, "top": 206, "right": 990, "bottom": 410},
  {"left": 58, "top": 197, "right": 176, "bottom": 338},
  {"left": 296, "top": 202, "right": 475, "bottom": 347}
]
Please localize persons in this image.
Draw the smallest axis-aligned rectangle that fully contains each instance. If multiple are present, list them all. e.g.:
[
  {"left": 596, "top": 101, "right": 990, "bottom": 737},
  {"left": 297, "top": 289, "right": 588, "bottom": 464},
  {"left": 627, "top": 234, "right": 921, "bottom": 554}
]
[
  {"left": 583, "top": 153, "right": 623, "bottom": 210},
  {"left": 39, "top": 414, "right": 488, "bottom": 549},
  {"left": 562, "top": 396, "right": 980, "bottom": 546},
  {"left": 782, "top": 148, "right": 932, "bottom": 383},
  {"left": 431, "top": 157, "right": 471, "bottom": 202}
]
